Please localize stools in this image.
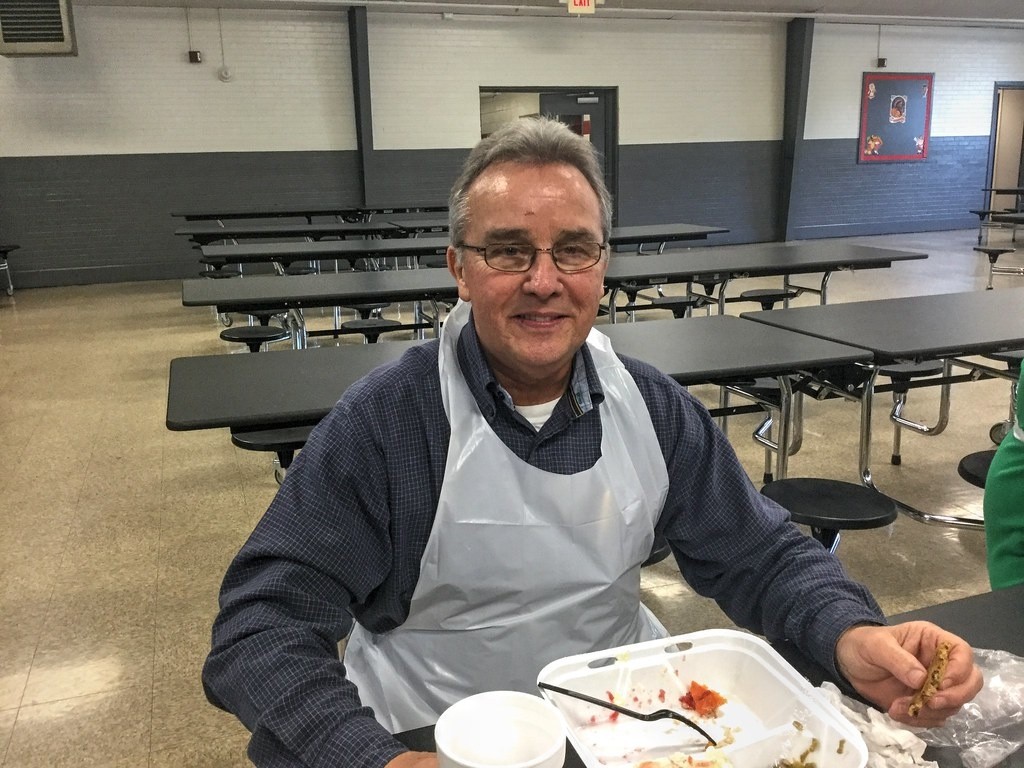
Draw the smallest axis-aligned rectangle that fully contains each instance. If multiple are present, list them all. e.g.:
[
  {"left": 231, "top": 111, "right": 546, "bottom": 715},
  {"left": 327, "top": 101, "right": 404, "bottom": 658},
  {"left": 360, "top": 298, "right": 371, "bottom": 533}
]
[
  {"left": 760, "top": 477, "right": 898, "bottom": 556},
  {"left": 0, "top": 244, "right": 21, "bottom": 296},
  {"left": 281, "top": 267, "right": 315, "bottom": 276},
  {"left": 1003, "top": 208, "right": 1024, "bottom": 243},
  {"left": 198, "top": 258, "right": 227, "bottom": 270},
  {"left": 218, "top": 325, "right": 285, "bottom": 352},
  {"left": 969, "top": 210, "right": 997, "bottom": 246},
  {"left": 426, "top": 260, "right": 448, "bottom": 268},
  {"left": 738, "top": 376, "right": 798, "bottom": 485},
  {"left": 237, "top": 309, "right": 289, "bottom": 353},
  {"left": 740, "top": 288, "right": 795, "bottom": 311},
  {"left": 199, "top": 269, "right": 242, "bottom": 279},
  {"left": 651, "top": 295, "right": 698, "bottom": 319},
  {"left": 618, "top": 285, "right": 651, "bottom": 323},
  {"left": 340, "top": 319, "right": 402, "bottom": 344},
  {"left": 639, "top": 534, "right": 671, "bottom": 570},
  {"left": 693, "top": 279, "right": 722, "bottom": 317},
  {"left": 973, "top": 247, "right": 1016, "bottom": 290},
  {"left": 353, "top": 264, "right": 392, "bottom": 272},
  {"left": 978, "top": 348, "right": 1024, "bottom": 447},
  {"left": 957, "top": 449, "right": 997, "bottom": 489},
  {"left": 229, "top": 419, "right": 317, "bottom": 486},
  {"left": 340, "top": 303, "right": 392, "bottom": 344},
  {"left": 877, "top": 358, "right": 945, "bottom": 465},
  {"left": 188, "top": 237, "right": 218, "bottom": 247}
]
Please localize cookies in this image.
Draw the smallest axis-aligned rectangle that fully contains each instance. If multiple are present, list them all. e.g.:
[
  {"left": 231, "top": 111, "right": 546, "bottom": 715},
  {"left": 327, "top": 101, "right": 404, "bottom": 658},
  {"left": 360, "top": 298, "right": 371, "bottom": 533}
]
[{"left": 907, "top": 642, "right": 950, "bottom": 719}]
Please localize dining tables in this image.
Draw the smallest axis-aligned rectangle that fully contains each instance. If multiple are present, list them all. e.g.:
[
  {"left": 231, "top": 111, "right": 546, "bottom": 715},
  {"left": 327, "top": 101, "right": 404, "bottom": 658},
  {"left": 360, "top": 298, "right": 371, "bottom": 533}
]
[{"left": 166, "top": 188, "right": 1024, "bottom": 768}]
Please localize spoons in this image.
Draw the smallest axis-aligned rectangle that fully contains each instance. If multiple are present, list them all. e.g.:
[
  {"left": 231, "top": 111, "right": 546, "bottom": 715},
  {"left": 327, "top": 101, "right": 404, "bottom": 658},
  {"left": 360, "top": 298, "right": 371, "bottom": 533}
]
[{"left": 537, "top": 683, "right": 719, "bottom": 747}]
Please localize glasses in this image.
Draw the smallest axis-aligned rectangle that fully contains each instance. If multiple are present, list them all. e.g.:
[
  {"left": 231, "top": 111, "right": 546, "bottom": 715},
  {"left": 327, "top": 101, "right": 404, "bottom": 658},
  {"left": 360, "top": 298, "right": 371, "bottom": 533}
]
[{"left": 455, "top": 237, "right": 609, "bottom": 273}]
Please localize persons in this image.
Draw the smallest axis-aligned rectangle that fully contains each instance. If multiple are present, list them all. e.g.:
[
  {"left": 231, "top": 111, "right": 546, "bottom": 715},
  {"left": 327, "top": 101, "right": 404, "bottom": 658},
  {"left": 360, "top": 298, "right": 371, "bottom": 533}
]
[
  {"left": 205, "top": 120, "right": 986, "bottom": 768},
  {"left": 984, "top": 352, "right": 1024, "bottom": 589}
]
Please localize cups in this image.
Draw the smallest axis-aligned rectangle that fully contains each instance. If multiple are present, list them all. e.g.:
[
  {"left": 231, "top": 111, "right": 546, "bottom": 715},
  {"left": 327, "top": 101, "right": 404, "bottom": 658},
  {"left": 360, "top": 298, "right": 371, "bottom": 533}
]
[{"left": 436, "top": 691, "right": 566, "bottom": 768}]
